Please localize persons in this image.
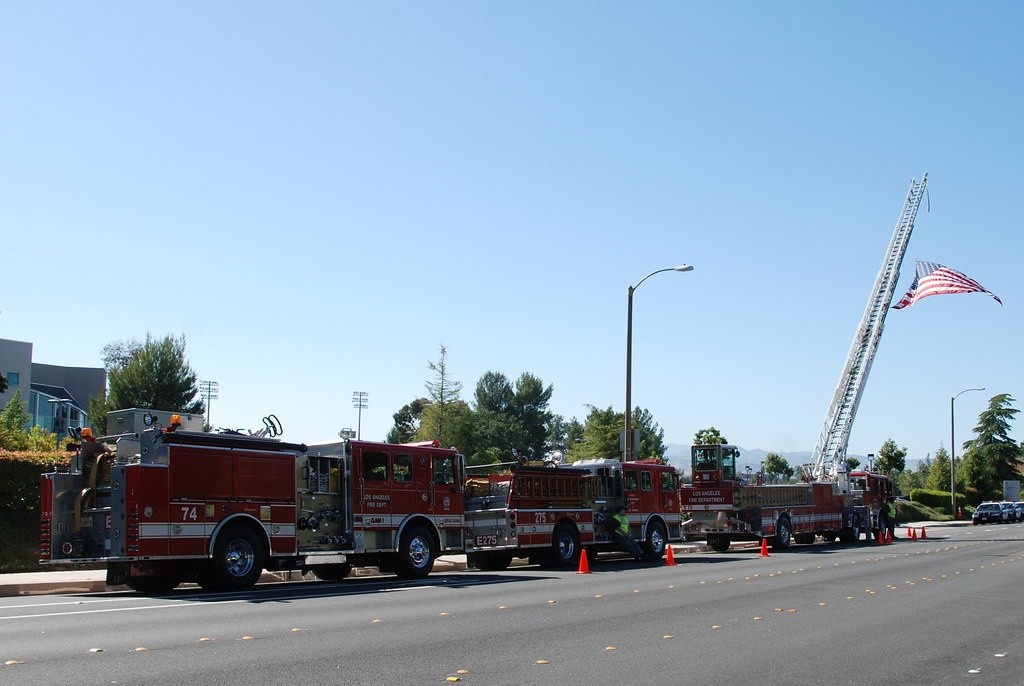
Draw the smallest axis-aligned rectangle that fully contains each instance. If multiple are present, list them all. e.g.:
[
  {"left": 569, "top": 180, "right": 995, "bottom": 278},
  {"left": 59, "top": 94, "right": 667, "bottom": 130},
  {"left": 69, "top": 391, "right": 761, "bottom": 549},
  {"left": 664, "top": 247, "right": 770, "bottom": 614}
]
[
  {"left": 610, "top": 505, "right": 652, "bottom": 563},
  {"left": 884, "top": 497, "right": 901, "bottom": 541}
]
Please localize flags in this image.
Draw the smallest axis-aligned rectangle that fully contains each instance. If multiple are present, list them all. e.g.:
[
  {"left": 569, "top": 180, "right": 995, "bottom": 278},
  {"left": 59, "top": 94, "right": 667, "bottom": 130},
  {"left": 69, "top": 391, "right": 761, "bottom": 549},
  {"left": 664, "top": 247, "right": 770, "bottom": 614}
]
[{"left": 890, "top": 259, "right": 1003, "bottom": 310}]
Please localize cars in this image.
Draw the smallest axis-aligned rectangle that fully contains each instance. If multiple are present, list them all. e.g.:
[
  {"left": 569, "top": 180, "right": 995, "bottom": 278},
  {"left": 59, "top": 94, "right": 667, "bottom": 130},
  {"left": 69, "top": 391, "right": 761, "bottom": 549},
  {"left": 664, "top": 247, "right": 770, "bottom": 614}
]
[
  {"left": 972, "top": 502, "right": 1003, "bottom": 525},
  {"left": 1014, "top": 502, "right": 1024, "bottom": 523},
  {"left": 998, "top": 501, "right": 1016, "bottom": 523}
]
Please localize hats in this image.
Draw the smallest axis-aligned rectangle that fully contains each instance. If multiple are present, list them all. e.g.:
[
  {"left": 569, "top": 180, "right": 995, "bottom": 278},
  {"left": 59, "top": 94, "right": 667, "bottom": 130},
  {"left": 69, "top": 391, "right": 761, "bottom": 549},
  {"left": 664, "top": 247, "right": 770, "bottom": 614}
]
[{"left": 616, "top": 505, "right": 627, "bottom": 514}]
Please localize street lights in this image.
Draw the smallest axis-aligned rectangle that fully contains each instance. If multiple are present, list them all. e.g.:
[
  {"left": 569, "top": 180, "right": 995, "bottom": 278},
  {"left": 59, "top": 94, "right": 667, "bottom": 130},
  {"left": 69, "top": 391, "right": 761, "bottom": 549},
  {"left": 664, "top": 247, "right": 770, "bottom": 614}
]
[
  {"left": 950, "top": 387, "right": 985, "bottom": 519},
  {"left": 623, "top": 265, "right": 695, "bottom": 463},
  {"left": 47, "top": 398, "right": 72, "bottom": 451}
]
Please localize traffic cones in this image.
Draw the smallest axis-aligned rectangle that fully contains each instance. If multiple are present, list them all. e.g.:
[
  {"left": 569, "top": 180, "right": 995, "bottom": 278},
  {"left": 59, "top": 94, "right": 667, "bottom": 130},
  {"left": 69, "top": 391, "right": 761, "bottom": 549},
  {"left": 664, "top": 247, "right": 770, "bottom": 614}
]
[
  {"left": 912, "top": 528, "right": 917, "bottom": 540},
  {"left": 665, "top": 545, "right": 676, "bottom": 566},
  {"left": 877, "top": 531, "right": 884, "bottom": 545},
  {"left": 907, "top": 526, "right": 911, "bottom": 536},
  {"left": 957, "top": 507, "right": 963, "bottom": 520},
  {"left": 758, "top": 538, "right": 770, "bottom": 557},
  {"left": 920, "top": 527, "right": 927, "bottom": 539},
  {"left": 886, "top": 530, "right": 892, "bottom": 544},
  {"left": 576, "top": 549, "right": 593, "bottom": 573}
]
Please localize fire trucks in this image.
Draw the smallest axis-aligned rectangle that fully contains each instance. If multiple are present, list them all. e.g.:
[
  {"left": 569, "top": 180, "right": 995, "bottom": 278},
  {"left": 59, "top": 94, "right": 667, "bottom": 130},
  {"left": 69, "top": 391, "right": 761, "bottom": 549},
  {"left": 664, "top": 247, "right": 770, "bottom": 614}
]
[
  {"left": 679, "top": 169, "right": 928, "bottom": 551},
  {"left": 39, "top": 408, "right": 467, "bottom": 592},
  {"left": 461, "top": 455, "right": 688, "bottom": 570}
]
